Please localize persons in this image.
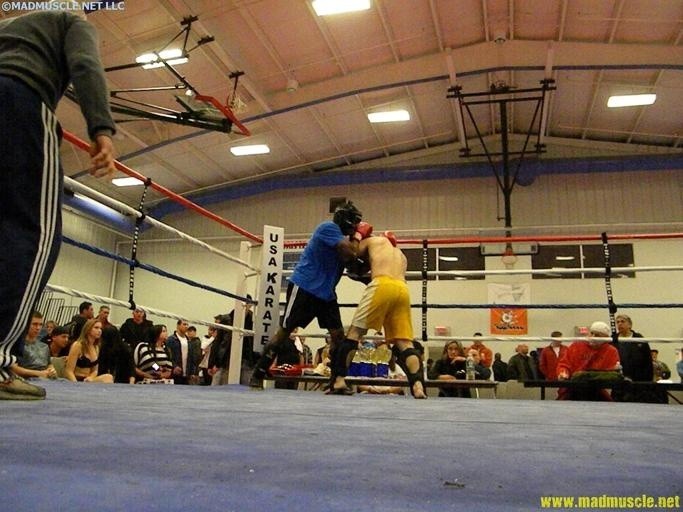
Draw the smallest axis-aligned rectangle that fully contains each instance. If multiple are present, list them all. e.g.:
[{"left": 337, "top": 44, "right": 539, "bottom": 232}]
[
  {"left": 322, "top": 232, "right": 427, "bottom": 399},
  {"left": 9, "top": 295, "right": 683, "bottom": 405},
  {"left": 250, "top": 204, "right": 396, "bottom": 392},
  {"left": 0, "top": 0, "right": 117, "bottom": 401}
]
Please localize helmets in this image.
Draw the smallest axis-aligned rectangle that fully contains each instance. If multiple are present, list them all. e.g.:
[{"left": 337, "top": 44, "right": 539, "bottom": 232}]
[{"left": 333, "top": 203, "right": 362, "bottom": 236}]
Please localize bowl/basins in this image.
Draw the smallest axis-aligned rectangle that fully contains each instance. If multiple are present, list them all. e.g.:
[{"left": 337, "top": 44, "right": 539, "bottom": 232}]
[{"left": 660, "top": 372, "right": 670, "bottom": 379}]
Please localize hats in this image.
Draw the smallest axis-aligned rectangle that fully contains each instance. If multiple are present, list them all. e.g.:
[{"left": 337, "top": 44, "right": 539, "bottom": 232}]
[{"left": 590, "top": 321, "right": 612, "bottom": 337}]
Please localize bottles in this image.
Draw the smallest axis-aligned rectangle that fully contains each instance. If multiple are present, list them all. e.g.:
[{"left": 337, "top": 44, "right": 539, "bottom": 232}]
[
  {"left": 655, "top": 364, "right": 660, "bottom": 376},
  {"left": 349, "top": 341, "right": 389, "bottom": 375},
  {"left": 464, "top": 351, "right": 475, "bottom": 380}
]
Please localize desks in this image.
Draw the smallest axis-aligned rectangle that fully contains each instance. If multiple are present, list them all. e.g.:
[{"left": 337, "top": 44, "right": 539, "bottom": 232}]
[
  {"left": 270, "top": 371, "right": 501, "bottom": 398},
  {"left": 524, "top": 379, "right": 683, "bottom": 404}
]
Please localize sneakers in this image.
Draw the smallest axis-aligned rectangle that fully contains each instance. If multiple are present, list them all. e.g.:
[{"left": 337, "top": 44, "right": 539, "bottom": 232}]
[{"left": 0, "top": 376, "right": 46, "bottom": 400}]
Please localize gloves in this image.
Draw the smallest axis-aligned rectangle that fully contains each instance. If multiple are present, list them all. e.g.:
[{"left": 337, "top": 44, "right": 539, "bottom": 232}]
[
  {"left": 357, "top": 222, "right": 373, "bottom": 239},
  {"left": 383, "top": 231, "right": 397, "bottom": 247}
]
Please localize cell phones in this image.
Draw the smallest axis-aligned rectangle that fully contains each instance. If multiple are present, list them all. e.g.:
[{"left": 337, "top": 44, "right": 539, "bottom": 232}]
[{"left": 151, "top": 361, "right": 160, "bottom": 371}]
